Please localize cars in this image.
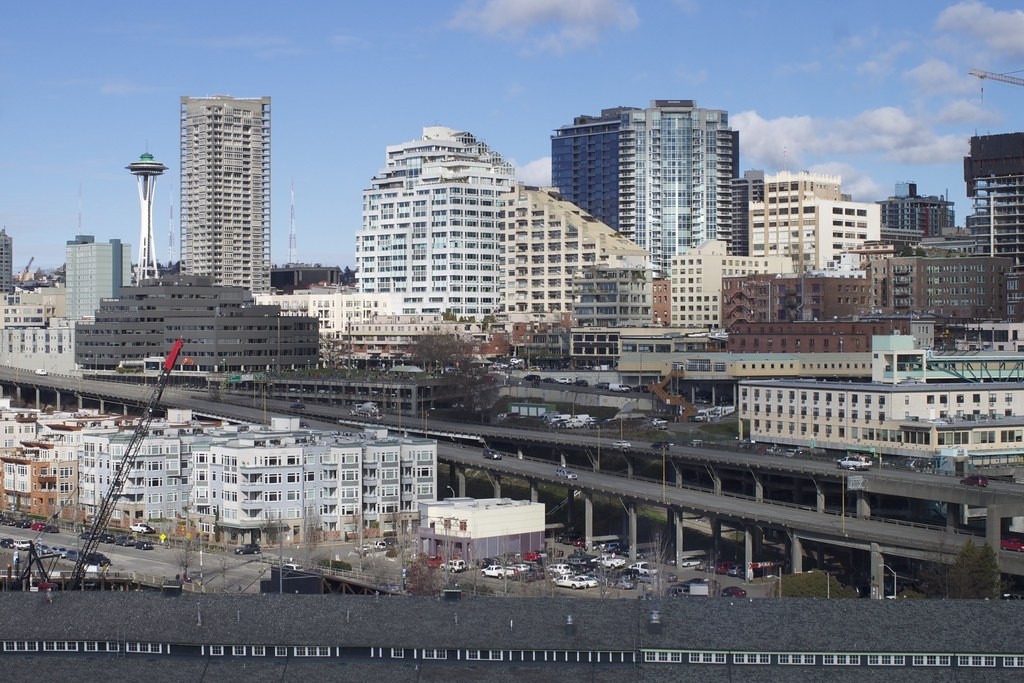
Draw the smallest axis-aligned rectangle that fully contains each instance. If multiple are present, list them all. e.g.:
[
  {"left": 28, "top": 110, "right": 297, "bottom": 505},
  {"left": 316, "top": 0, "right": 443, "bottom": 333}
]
[
  {"left": 35, "top": 368, "right": 49, "bottom": 375},
  {"left": 301, "top": 421, "right": 311, "bottom": 428},
  {"left": 0, "top": 537, "right": 112, "bottom": 566},
  {"left": 740, "top": 443, "right": 934, "bottom": 469},
  {"left": 488, "top": 358, "right": 542, "bottom": 371},
  {"left": 523, "top": 374, "right": 648, "bottom": 392},
  {"left": 1001, "top": 538, "right": 1024, "bottom": 552},
  {"left": 836, "top": 456, "right": 872, "bottom": 471},
  {"left": 427, "top": 530, "right": 747, "bottom": 598},
  {"left": 57, "top": 565, "right": 59, "bottom": 566},
  {"left": 355, "top": 537, "right": 398, "bottom": 552},
  {"left": 0, "top": 514, "right": 154, "bottom": 550},
  {"left": 959, "top": 474, "right": 988, "bottom": 487}
]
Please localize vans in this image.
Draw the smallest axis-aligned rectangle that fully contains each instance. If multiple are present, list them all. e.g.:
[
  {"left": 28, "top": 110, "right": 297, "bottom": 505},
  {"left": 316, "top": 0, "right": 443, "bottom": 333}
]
[{"left": 282, "top": 564, "right": 303, "bottom": 571}]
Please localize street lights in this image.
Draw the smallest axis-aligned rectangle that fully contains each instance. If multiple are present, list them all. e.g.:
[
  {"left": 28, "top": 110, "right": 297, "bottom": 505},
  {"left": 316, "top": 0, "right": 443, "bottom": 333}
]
[
  {"left": 878, "top": 563, "right": 897, "bottom": 600},
  {"left": 1003, "top": 594, "right": 1023, "bottom": 599},
  {"left": 808, "top": 569, "right": 830, "bottom": 598},
  {"left": 422, "top": 385, "right": 431, "bottom": 430},
  {"left": 767, "top": 574, "right": 783, "bottom": 598}
]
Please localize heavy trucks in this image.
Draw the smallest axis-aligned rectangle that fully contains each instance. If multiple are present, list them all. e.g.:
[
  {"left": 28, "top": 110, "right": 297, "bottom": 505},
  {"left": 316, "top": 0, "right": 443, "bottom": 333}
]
[
  {"left": 653, "top": 418, "right": 669, "bottom": 430},
  {"left": 695, "top": 405, "right": 735, "bottom": 421},
  {"left": 349, "top": 401, "right": 385, "bottom": 419}
]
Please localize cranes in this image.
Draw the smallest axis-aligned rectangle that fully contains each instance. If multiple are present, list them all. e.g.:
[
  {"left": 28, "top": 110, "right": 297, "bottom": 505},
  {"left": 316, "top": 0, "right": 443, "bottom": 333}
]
[{"left": 967, "top": 67, "right": 1024, "bottom": 111}]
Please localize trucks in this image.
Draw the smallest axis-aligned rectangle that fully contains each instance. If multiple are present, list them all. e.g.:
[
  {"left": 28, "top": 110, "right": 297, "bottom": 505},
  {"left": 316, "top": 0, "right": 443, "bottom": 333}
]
[{"left": 540, "top": 408, "right": 599, "bottom": 428}]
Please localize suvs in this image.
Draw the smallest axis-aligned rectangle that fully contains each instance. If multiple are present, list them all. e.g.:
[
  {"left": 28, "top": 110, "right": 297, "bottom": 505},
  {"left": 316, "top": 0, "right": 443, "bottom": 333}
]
[
  {"left": 555, "top": 469, "right": 578, "bottom": 480},
  {"left": 650, "top": 440, "right": 670, "bottom": 451},
  {"left": 483, "top": 449, "right": 501, "bottom": 459},
  {"left": 289, "top": 402, "right": 307, "bottom": 411},
  {"left": 235, "top": 543, "right": 261, "bottom": 555},
  {"left": 611, "top": 440, "right": 631, "bottom": 448}
]
